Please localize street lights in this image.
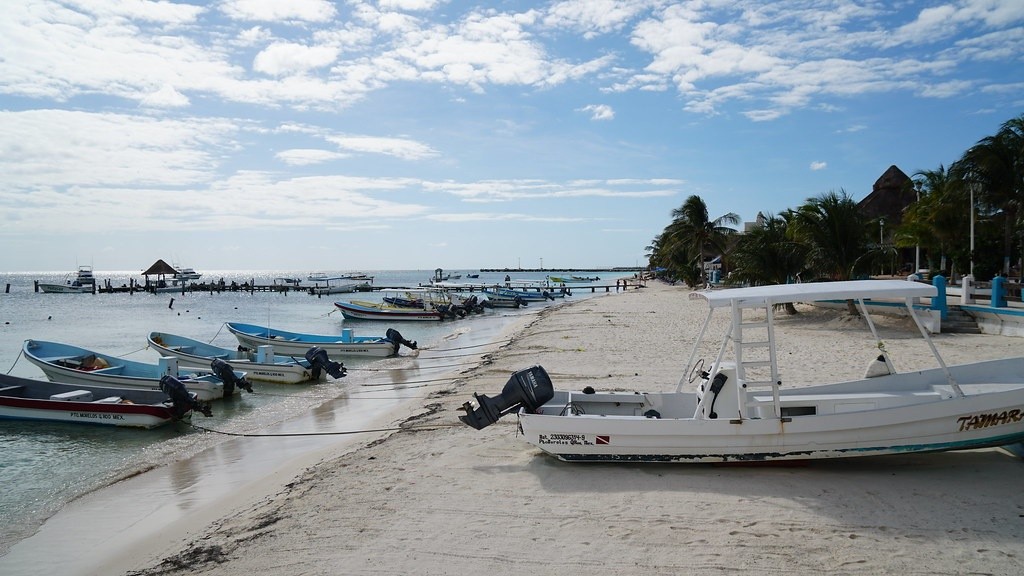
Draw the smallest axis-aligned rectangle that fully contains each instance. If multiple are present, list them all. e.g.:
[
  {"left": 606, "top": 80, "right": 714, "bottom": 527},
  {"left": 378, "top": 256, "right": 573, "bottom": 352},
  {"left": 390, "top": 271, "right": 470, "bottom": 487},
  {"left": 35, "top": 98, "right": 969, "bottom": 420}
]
[
  {"left": 964, "top": 172, "right": 979, "bottom": 276},
  {"left": 878, "top": 218, "right": 886, "bottom": 275},
  {"left": 913, "top": 180, "right": 925, "bottom": 275}
]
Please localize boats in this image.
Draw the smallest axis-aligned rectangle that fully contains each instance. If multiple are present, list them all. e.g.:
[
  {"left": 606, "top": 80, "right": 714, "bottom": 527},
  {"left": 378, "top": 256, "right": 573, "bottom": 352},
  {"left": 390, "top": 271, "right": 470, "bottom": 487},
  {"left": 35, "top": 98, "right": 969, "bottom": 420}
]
[
  {"left": 461, "top": 280, "right": 1024, "bottom": 469},
  {"left": 222, "top": 320, "right": 422, "bottom": 358},
  {"left": 175, "top": 266, "right": 202, "bottom": 280},
  {"left": 308, "top": 275, "right": 354, "bottom": 295},
  {"left": 23, "top": 338, "right": 255, "bottom": 407},
  {"left": 0, "top": 373, "right": 216, "bottom": 431},
  {"left": 40, "top": 272, "right": 97, "bottom": 295},
  {"left": 144, "top": 329, "right": 346, "bottom": 386},
  {"left": 332, "top": 274, "right": 605, "bottom": 323}
]
